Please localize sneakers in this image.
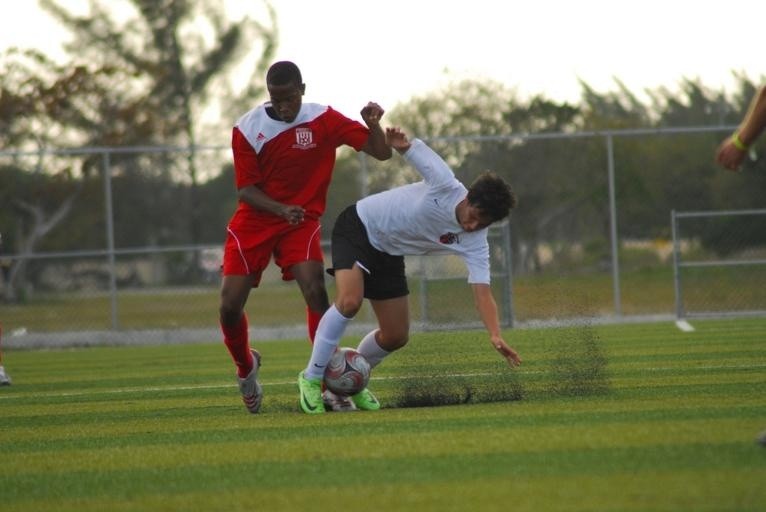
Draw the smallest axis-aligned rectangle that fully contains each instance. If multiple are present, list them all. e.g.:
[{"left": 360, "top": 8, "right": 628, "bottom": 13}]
[
  {"left": 236, "top": 348, "right": 262, "bottom": 414},
  {"left": 352, "top": 388, "right": 379, "bottom": 412},
  {"left": 322, "top": 389, "right": 356, "bottom": 413},
  {"left": 297, "top": 369, "right": 324, "bottom": 415}
]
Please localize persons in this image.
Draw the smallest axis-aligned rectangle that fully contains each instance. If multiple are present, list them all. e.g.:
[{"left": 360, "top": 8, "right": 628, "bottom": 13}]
[
  {"left": 297, "top": 126, "right": 522, "bottom": 414},
  {"left": 218, "top": 62, "right": 391, "bottom": 412},
  {"left": 718, "top": 84, "right": 765, "bottom": 170}
]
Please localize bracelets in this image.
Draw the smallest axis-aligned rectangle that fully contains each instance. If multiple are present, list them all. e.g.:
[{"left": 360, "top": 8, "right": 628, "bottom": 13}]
[{"left": 731, "top": 132, "right": 746, "bottom": 151}]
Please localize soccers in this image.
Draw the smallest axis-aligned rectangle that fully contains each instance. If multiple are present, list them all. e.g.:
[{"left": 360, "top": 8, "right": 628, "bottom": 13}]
[{"left": 323, "top": 346, "right": 370, "bottom": 397}]
